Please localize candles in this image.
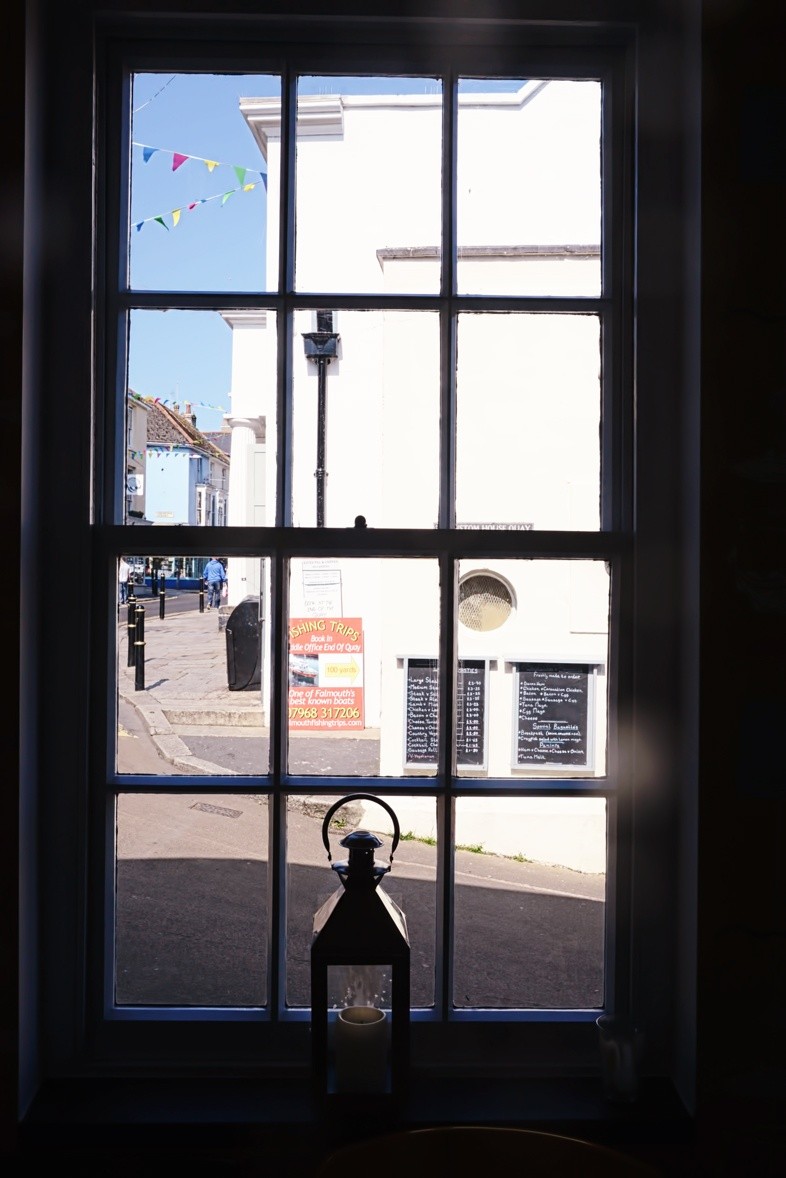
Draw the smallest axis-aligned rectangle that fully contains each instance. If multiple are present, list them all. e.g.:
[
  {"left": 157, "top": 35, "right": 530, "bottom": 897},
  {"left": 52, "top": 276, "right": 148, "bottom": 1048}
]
[{"left": 338, "top": 1004, "right": 390, "bottom": 1098}]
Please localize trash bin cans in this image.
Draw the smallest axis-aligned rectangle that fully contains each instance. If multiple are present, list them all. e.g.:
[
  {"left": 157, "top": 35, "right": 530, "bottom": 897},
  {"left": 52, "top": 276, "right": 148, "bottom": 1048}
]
[{"left": 224, "top": 595, "right": 261, "bottom": 691}]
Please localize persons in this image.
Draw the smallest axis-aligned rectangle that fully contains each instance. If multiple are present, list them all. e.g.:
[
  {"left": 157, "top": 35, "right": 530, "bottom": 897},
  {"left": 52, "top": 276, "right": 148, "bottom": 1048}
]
[
  {"left": 202, "top": 558, "right": 226, "bottom": 609},
  {"left": 118, "top": 555, "right": 130, "bottom": 604}
]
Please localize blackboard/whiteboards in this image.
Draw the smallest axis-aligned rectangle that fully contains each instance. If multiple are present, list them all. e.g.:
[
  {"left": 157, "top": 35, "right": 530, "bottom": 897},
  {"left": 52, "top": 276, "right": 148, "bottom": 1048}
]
[
  {"left": 510, "top": 662, "right": 596, "bottom": 770},
  {"left": 404, "top": 657, "right": 489, "bottom": 770}
]
